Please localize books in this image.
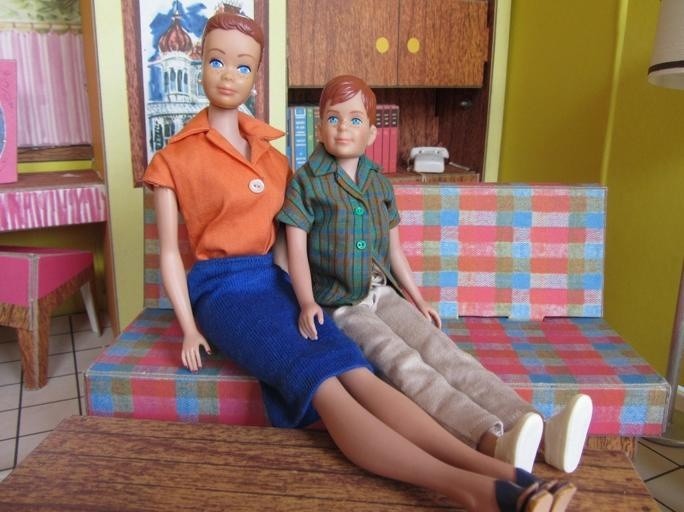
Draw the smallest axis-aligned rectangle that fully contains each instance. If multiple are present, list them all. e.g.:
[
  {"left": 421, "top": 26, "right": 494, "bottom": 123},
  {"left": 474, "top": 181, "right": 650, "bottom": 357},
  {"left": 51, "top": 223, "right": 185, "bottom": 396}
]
[{"left": 286, "top": 101, "right": 400, "bottom": 176}]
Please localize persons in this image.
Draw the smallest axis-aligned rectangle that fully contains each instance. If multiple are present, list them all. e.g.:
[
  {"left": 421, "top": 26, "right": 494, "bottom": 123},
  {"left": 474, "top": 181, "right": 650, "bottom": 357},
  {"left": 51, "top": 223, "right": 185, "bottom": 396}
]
[
  {"left": 141, "top": 13, "right": 579, "bottom": 512},
  {"left": 271, "top": 74, "right": 594, "bottom": 477}
]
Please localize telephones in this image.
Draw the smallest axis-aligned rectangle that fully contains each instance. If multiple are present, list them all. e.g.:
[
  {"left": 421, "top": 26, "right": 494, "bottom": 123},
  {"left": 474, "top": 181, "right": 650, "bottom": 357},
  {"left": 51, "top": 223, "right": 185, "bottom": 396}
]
[{"left": 405, "top": 146, "right": 449, "bottom": 174}]
